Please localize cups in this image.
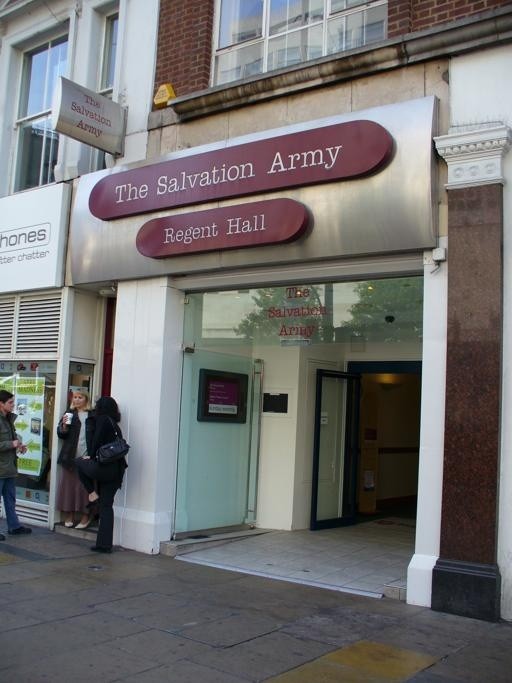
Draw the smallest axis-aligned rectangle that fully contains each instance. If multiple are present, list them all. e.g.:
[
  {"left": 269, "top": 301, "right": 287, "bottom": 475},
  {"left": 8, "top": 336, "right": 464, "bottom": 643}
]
[{"left": 66, "top": 412, "right": 73, "bottom": 424}]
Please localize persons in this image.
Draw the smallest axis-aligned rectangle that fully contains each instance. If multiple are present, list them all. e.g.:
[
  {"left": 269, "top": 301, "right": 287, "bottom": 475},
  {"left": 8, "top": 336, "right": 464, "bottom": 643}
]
[
  {"left": 55, "top": 389, "right": 96, "bottom": 529},
  {"left": 0, "top": 390, "right": 32, "bottom": 541},
  {"left": 76, "top": 396, "right": 129, "bottom": 553}
]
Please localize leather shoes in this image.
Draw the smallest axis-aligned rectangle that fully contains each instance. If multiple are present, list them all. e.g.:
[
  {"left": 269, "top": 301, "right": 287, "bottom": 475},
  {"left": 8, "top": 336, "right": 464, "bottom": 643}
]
[
  {"left": 75, "top": 516, "right": 93, "bottom": 529},
  {"left": 64, "top": 520, "right": 74, "bottom": 527},
  {"left": 86, "top": 497, "right": 101, "bottom": 508},
  {"left": 92, "top": 545, "right": 111, "bottom": 553}
]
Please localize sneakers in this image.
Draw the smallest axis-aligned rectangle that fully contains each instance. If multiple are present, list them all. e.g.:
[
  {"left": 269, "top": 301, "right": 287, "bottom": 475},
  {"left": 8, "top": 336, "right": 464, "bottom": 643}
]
[
  {"left": 0, "top": 534, "right": 5, "bottom": 540},
  {"left": 9, "top": 526, "right": 32, "bottom": 534}
]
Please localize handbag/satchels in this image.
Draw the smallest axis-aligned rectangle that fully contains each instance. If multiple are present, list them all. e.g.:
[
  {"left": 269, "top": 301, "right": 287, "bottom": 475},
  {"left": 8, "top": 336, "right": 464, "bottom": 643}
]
[{"left": 96, "top": 439, "right": 129, "bottom": 463}]
